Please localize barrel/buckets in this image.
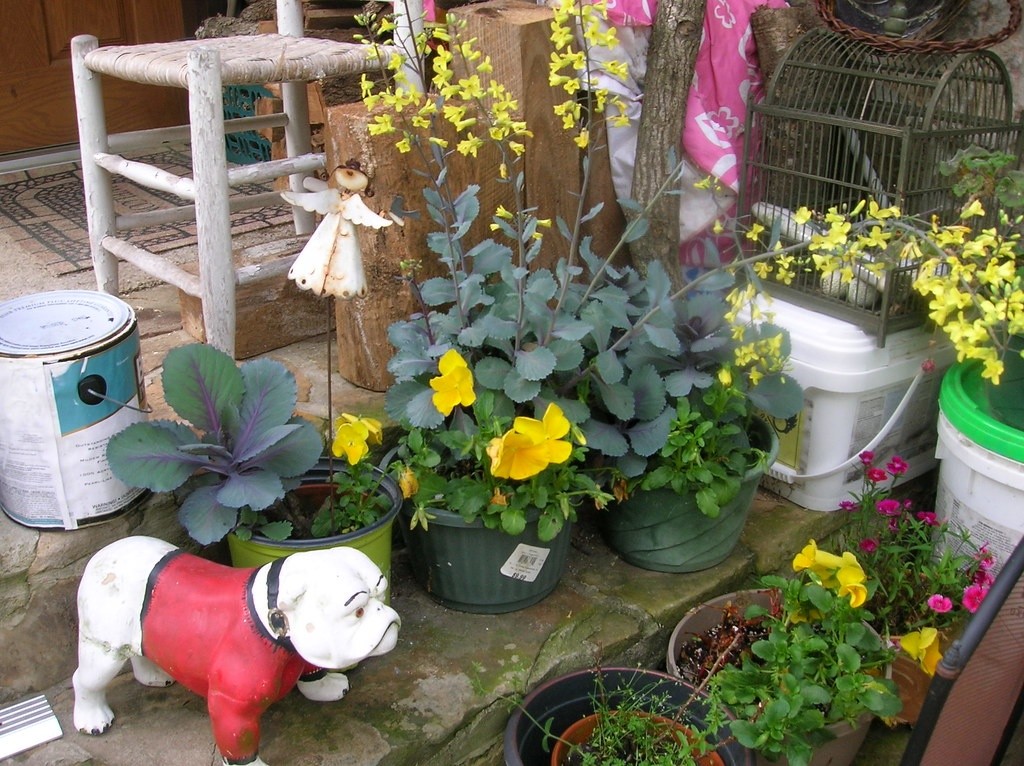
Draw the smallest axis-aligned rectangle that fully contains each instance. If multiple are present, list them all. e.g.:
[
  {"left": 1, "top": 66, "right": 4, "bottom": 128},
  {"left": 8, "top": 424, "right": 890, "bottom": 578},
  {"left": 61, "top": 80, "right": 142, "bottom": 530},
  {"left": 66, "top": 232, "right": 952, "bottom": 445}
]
[
  {"left": 929, "top": 338, "right": 1024, "bottom": 584},
  {"left": 0, "top": 290, "right": 153, "bottom": 532}
]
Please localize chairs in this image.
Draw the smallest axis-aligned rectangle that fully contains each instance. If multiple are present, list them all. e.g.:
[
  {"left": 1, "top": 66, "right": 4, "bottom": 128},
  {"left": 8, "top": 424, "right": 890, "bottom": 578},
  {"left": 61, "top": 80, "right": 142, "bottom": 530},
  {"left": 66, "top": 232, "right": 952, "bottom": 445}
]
[{"left": 71, "top": 0, "right": 426, "bottom": 364}]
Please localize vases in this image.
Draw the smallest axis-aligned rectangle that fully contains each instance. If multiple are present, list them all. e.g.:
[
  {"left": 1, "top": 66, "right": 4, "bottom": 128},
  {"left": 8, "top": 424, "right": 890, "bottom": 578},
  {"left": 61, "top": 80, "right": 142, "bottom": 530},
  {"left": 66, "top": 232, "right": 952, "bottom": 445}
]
[
  {"left": 877, "top": 568, "right": 970, "bottom": 732},
  {"left": 502, "top": 665, "right": 757, "bottom": 766},
  {"left": 229, "top": 456, "right": 404, "bottom": 674},
  {"left": 380, "top": 446, "right": 590, "bottom": 616},
  {"left": 550, "top": 710, "right": 726, "bottom": 766},
  {"left": 601, "top": 405, "right": 781, "bottom": 574},
  {"left": 665, "top": 588, "right": 893, "bottom": 766}
]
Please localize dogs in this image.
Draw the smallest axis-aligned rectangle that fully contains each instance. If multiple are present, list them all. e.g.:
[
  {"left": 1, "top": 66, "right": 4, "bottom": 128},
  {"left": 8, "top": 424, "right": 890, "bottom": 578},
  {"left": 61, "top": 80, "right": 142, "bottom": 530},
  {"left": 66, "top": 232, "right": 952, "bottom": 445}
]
[{"left": 71, "top": 533, "right": 403, "bottom": 766}]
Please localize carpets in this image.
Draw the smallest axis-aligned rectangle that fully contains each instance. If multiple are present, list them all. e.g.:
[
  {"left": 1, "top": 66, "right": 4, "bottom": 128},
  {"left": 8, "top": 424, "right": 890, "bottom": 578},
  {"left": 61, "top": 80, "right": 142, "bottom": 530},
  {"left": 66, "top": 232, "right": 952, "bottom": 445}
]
[{"left": 0, "top": 138, "right": 294, "bottom": 278}]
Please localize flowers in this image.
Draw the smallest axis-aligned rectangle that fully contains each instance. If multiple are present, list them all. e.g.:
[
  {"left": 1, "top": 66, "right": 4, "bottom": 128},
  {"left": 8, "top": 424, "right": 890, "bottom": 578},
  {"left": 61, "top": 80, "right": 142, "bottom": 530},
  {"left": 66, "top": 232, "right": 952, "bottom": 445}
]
[
  {"left": 836, "top": 449, "right": 997, "bottom": 634},
  {"left": 651, "top": 537, "right": 944, "bottom": 766},
  {"left": 352, "top": 347, "right": 629, "bottom": 537},
  {"left": 356, "top": 0, "right": 1024, "bottom": 387},
  {"left": 323, "top": 410, "right": 419, "bottom": 528},
  {"left": 705, "top": 364, "right": 747, "bottom": 472}
]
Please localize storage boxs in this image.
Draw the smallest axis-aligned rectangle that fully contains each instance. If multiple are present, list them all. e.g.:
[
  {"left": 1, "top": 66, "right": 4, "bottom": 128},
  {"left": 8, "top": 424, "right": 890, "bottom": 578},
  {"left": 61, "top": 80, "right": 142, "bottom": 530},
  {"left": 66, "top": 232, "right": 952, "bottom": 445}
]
[{"left": 727, "top": 290, "right": 979, "bottom": 511}]
